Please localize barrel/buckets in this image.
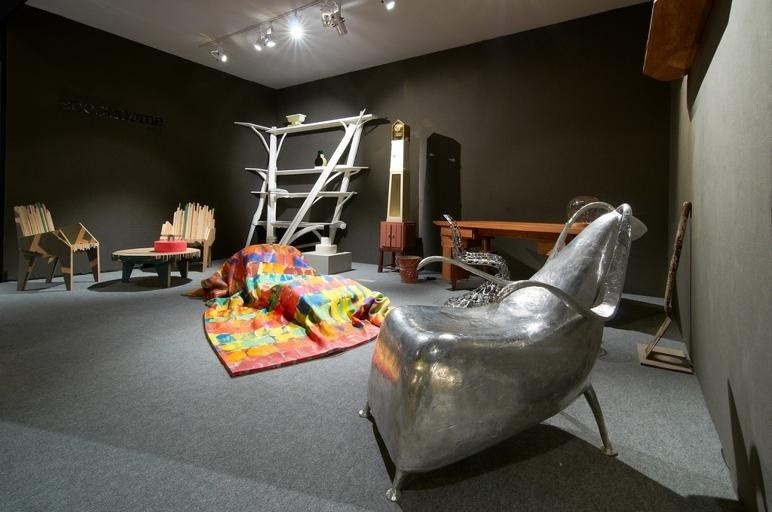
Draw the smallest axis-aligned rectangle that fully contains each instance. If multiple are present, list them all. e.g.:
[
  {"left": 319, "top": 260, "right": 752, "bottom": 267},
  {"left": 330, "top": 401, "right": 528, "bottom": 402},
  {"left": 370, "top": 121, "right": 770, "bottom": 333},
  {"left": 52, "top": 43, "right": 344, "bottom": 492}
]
[{"left": 395, "top": 255, "right": 421, "bottom": 284}]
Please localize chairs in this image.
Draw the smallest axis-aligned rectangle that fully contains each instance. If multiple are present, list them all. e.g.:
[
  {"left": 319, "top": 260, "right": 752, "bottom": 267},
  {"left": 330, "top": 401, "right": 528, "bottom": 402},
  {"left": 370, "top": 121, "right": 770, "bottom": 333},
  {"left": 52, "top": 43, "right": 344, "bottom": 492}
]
[
  {"left": 358, "top": 201, "right": 633, "bottom": 502},
  {"left": 13, "top": 202, "right": 100, "bottom": 291},
  {"left": 442, "top": 214, "right": 510, "bottom": 309},
  {"left": 159, "top": 202, "right": 216, "bottom": 272}
]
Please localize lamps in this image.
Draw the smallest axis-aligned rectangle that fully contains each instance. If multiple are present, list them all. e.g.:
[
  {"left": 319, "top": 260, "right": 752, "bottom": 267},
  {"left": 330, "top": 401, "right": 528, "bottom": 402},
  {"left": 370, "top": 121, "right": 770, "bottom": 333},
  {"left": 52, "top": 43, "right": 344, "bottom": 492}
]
[{"left": 197, "top": 0, "right": 395, "bottom": 64}]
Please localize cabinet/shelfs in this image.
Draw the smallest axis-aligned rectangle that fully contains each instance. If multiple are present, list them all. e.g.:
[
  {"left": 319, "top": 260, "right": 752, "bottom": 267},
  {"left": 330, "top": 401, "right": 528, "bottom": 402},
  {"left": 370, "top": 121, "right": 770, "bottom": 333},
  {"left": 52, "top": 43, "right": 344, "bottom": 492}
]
[
  {"left": 233, "top": 108, "right": 373, "bottom": 247},
  {"left": 378, "top": 220, "right": 416, "bottom": 272}
]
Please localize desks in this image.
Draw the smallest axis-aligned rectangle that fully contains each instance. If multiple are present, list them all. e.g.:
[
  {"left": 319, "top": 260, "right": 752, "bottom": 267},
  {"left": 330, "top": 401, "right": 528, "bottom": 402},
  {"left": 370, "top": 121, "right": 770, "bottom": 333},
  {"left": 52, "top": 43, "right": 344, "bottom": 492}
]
[
  {"left": 432, "top": 219, "right": 592, "bottom": 290},
  {"left": 112, "top": 247, "right": 201, "bottom": 288}
]
